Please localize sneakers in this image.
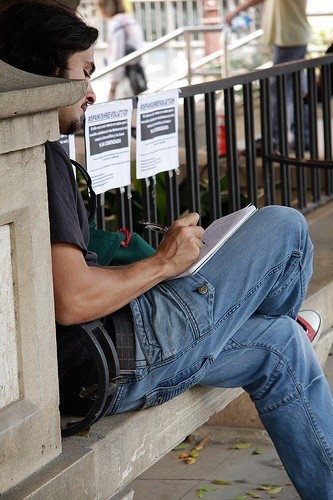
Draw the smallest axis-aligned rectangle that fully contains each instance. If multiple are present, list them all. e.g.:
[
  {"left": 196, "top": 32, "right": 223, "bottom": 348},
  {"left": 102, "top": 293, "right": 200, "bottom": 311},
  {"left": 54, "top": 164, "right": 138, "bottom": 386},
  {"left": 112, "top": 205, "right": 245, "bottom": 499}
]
[{"left": 296, "top": 308, "right": 324, "bottom": 348}]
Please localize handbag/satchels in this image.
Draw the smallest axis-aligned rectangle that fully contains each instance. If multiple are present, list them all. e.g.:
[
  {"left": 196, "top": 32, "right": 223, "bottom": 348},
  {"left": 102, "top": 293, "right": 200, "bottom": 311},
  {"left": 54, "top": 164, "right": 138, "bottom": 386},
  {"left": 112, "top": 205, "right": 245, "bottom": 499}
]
[
  {"left": 64, "top": 158, "right": 157, "bottom": 268},
  {"left": 125, "top": 63, "right": 148, "bottom": 95}
]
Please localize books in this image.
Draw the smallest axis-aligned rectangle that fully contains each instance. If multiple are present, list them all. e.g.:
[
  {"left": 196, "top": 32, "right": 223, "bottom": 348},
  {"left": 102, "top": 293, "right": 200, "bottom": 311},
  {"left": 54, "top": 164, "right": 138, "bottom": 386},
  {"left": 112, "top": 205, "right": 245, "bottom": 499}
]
[{"left": 158, "top": 203, "right": 258, "bottom": 283}]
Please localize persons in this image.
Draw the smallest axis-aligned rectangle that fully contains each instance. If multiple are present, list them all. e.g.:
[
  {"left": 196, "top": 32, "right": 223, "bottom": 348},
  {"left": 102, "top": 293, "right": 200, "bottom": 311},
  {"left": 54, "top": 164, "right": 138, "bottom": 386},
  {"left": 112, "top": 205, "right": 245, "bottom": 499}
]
[
  {"left": 226, "top": 1, "right": 314, "bottom": 154},
  {"left": 0, "top": 0, "right": 333, "bottom": 500},
  {"left": 97, "top": 0, "right": 147, "bottom": 101}
]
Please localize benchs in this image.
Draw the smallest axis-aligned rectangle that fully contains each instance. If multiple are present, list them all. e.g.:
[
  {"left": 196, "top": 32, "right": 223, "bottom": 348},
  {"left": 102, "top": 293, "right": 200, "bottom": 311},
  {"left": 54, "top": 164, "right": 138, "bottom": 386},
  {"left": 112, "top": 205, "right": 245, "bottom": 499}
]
[{"left": 0, "top": 59, "right": 333, "bottom": 500}]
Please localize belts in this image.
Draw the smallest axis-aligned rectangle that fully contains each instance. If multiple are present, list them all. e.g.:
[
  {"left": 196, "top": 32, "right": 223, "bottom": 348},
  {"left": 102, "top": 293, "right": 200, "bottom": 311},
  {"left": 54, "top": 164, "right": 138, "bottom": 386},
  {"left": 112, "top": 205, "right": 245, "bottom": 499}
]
[{"left": 112, "top": 304, "right": 139, "bottom": 372}]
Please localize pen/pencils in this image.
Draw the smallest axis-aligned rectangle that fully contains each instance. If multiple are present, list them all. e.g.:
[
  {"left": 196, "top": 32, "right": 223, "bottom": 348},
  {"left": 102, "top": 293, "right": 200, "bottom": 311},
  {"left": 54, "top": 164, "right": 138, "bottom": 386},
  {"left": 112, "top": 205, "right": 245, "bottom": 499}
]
[{"left": 138, "top": 219, "right": 208, "bottom": 246}]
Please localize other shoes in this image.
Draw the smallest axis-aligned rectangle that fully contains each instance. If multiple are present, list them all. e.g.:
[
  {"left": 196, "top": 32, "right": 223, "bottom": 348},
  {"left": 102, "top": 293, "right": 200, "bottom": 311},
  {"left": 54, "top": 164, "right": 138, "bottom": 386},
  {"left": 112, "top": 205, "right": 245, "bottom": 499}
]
[{"left": 290, "top": 142, "right": 312, "bottom": 155}]
